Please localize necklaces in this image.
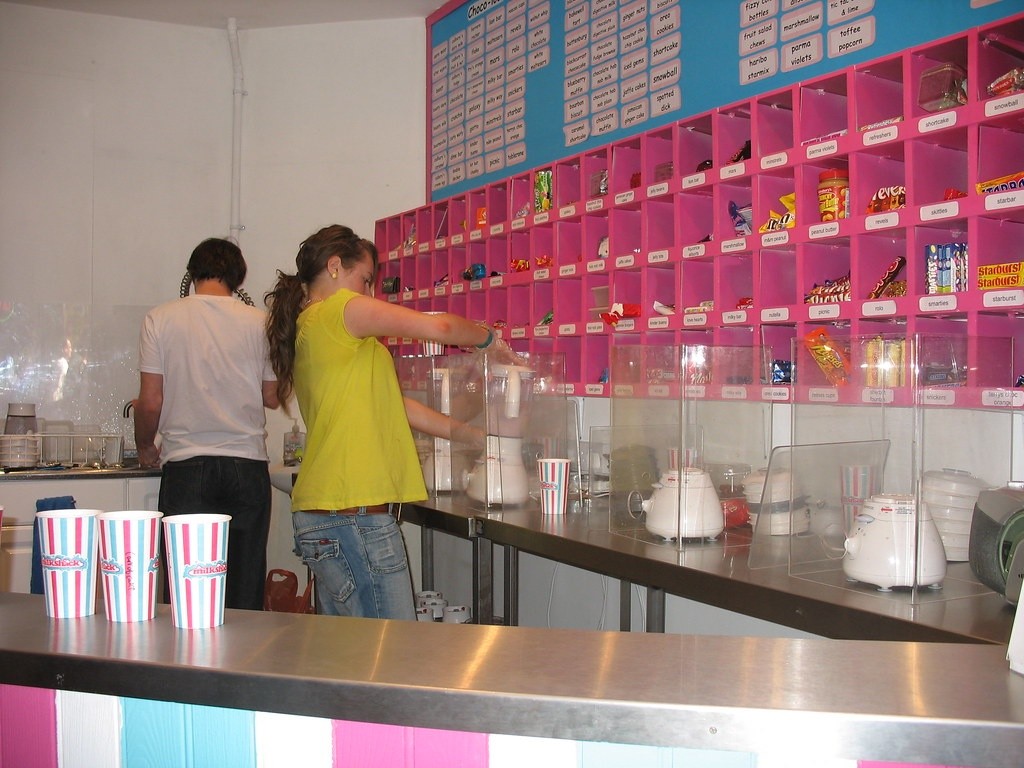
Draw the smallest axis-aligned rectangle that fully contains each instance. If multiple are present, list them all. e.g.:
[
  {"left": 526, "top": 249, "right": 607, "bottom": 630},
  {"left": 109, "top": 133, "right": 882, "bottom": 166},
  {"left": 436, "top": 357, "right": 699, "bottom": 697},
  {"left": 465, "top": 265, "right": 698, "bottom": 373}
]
[{"left": 302, "top": 297, "right": 323, "bottom": 307}]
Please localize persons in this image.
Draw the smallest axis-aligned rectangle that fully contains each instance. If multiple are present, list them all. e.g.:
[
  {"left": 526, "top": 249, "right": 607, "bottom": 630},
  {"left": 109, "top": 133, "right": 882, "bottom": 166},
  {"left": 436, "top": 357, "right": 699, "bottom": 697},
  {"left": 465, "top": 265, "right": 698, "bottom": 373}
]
[
  {"left": 133, "top": 238, "right": 280, "bottom": 611},
  {"left": 267, "top": 224, "right": 523, "bottom": 622}
]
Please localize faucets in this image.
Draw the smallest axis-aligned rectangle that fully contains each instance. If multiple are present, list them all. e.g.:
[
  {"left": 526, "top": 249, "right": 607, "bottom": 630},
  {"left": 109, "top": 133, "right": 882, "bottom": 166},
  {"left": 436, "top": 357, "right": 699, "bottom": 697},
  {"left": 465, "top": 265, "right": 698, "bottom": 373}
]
[{"left": 122, "top": 399, "right": 135, "bottom": 418}]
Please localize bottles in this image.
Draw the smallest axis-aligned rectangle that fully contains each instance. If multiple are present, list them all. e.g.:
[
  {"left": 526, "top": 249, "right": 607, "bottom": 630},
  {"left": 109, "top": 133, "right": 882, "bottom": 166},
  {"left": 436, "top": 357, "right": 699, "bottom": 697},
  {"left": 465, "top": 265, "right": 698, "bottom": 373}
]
[{"left": 590, "top": 169, "right": 608, "bottom": 198}]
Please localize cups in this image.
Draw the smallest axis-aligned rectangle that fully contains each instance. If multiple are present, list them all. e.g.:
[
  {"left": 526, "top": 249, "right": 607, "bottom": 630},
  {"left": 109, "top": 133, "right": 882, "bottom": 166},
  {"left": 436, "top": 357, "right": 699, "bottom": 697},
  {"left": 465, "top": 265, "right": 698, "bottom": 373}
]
[
  {"left": 420, "top": 311, "right": 450, "bottom": 357},
  {"left": 835, "top": 463, "right": 876, "bottom": 537},
  {"left": 161, "top": 514, "right": 232, "bottom": 629},
  {"left": 34, "top": 508, "right": 104, "bottom": 619},
  {"left": 537, "top": 458, "right": 572, "bottom": 515},
  {"left": 668, "top": 448, "right": 694, "bottom": 469},
  {"left": 98, "top": 511, "right": 164, "bottom": 623}
]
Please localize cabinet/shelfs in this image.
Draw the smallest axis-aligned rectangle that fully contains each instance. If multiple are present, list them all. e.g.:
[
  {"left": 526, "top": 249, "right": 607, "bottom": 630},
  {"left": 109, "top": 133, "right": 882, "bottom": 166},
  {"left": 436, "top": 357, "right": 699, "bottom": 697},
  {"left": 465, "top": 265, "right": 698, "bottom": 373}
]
[
  {"left": 0, "top": 477, "right": 164, "bottom": 605},
  {"left": 373, "top": 11, "right": 1023, "bottom": 413}
]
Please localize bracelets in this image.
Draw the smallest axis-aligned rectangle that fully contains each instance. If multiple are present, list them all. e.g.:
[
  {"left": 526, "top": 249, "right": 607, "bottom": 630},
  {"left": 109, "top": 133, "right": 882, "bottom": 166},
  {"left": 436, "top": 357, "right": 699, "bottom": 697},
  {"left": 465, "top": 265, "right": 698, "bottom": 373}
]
[{"left": 475, "top": 325, "right": 493, "bottom": 350}]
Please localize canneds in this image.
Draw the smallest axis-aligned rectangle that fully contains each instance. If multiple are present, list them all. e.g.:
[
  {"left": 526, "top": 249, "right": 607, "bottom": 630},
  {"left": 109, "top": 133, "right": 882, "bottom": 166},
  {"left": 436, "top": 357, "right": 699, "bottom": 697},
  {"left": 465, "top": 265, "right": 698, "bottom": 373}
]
[{"left": 816, "top": 169, "right": 849, "bottom": 222}]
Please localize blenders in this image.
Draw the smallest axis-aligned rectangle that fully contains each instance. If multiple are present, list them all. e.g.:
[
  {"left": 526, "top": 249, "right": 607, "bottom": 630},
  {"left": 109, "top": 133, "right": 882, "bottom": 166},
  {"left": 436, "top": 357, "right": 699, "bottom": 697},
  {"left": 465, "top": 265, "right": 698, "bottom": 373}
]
[
  {"left": 421, "top": 367, "right": 482, "bottom": 493},
  {"left": 644, "top": 468, "right": 725, "bottom": 542},
  {"left": 465, "top": 362, "right": 535, "bottom": 505},
  {"left": 4, "top": 402, "right": 38, "bottom": 470},
  {"left": 842, "top": 489, "right": 948, "bottom": 593}
]
